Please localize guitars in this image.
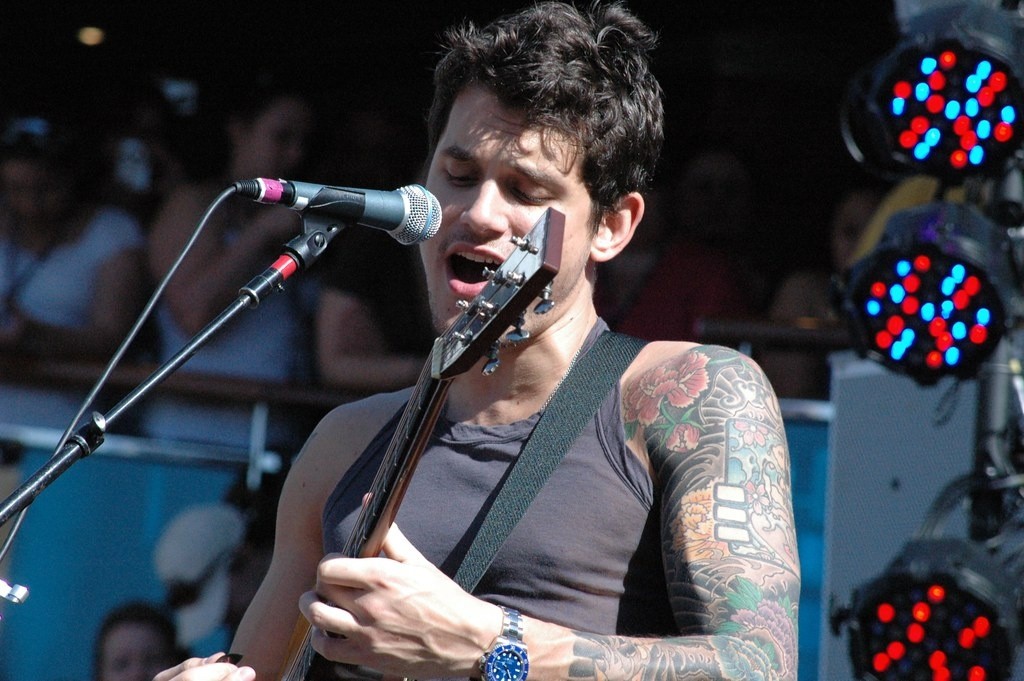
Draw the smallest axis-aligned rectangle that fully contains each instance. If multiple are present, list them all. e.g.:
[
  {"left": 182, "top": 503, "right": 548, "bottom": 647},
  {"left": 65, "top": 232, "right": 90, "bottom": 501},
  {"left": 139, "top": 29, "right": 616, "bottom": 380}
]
[{"left": 277, "top": 206, "right": 566, "bottom": 681}]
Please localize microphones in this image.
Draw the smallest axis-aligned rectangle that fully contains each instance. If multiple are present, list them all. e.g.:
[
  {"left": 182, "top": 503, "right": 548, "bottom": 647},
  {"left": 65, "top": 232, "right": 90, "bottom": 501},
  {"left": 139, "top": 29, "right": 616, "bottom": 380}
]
[{"left": 232, "top": 177, "right": 442, "bottom": 247}]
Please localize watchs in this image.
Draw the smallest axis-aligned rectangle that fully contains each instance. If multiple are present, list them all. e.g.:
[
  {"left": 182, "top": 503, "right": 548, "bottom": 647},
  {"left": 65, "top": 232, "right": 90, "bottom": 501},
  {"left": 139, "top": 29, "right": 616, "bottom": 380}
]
[{"left": 478, "top": 605, "right": 530, "bottom": 681}]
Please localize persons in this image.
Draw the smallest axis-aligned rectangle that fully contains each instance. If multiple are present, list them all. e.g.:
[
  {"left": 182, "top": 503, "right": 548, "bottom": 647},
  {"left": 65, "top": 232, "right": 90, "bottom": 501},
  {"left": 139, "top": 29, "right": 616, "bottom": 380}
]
[
  {"left": 0, "top": 52, "right": 891, "bottom": 404},
  {"left": 151, "top": 0, "right": 802, "bottom": 681},
  {"left": 94, "top": 603, "right": 177, "bottom": 681}
]
